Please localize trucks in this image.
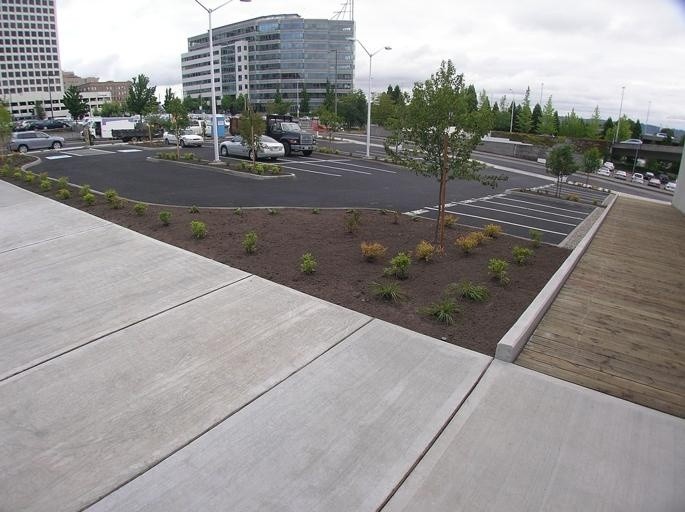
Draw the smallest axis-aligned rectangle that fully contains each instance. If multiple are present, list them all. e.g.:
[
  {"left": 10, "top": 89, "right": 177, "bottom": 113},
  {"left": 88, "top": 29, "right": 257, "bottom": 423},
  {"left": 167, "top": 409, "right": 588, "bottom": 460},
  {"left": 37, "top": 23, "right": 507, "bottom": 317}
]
[{"left": 230, "top": 114, "right": 316, "bottom": 158}]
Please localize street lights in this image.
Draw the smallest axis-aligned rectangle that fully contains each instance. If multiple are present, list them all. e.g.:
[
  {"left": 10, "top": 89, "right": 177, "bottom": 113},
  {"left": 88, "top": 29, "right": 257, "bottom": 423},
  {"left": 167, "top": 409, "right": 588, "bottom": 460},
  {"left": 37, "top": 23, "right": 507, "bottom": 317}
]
[
  {"left": 346, "top": 36, "right": 392, "bottom": 156},
  {"left": 196, "top": 0, "right": 251, "bottom": 162}
]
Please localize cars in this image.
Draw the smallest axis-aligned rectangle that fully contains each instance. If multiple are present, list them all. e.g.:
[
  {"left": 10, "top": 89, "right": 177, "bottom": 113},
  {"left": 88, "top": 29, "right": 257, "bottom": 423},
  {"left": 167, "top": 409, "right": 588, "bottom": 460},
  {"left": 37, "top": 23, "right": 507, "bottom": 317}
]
[
  {"left": 8, "top": 131, "right": 65, "bottom": 152},
  {"left": 620, "top": 139, "right": 642, "bottom": 144},
  {"left": 9, "top": 114, "right": 231, "bottom": 136},
  {"left": 598, "top": 158, "right": 676, "bottom": 192},
  {"left": 163, "top": 129, "right": 204, "bottom": 148},
  {"left": 220, "top": 134, "right": 285, "bottom": 162},
  {"left": 656, "top": 133, "right": 675, "bottom": 140}
]
